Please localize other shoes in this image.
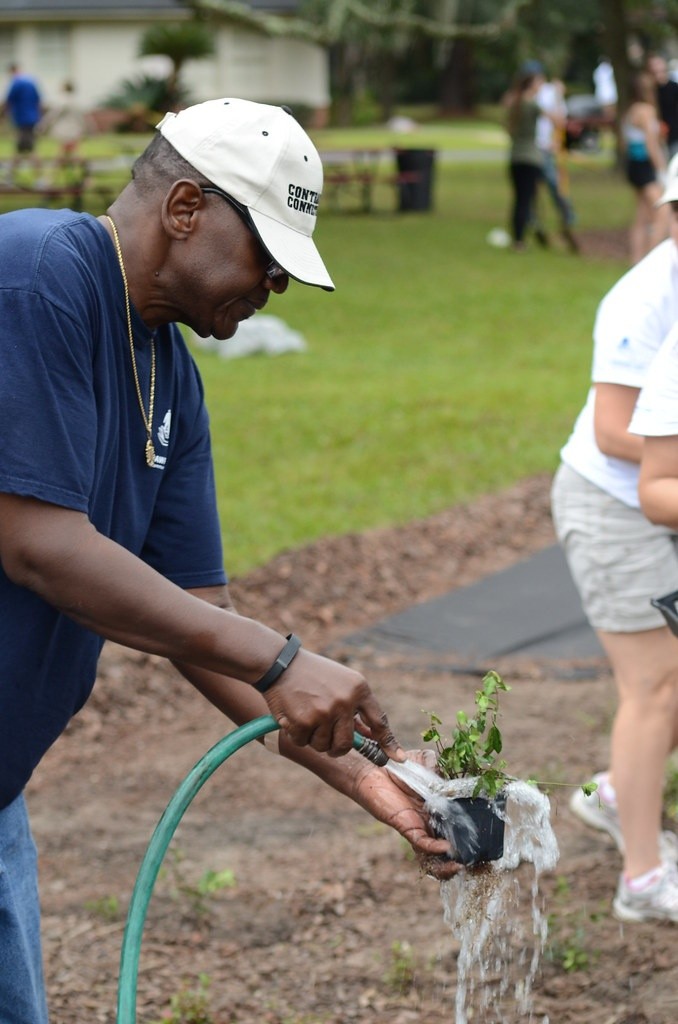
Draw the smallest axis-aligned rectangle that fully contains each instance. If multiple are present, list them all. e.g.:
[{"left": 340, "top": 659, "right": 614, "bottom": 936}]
[
  {"left": 561, "top": 227, "right": 579, "bottom": 252},
  {"left": 513, "top": 241, "right": 527, "bottom": 252},
  {"left": 535, "top": 229, "right": 547, "bottom": 249}
]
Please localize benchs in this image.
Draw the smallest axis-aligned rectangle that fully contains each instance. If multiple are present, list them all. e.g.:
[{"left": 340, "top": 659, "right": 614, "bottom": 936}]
[
  {"left": 1, "top": 156, "right": 107, "bottom": 211},
  {"left": 324, "top": 144, "right": 421, "bottom": 211}
]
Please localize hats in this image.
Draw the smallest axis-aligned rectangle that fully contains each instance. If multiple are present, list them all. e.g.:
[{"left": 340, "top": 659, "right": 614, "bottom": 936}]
[
  {"left": 515, "top": 58, "right": 544, "bottom": 81},
  {"left": 654, "top": 153, "right": 678, "bottom": 208},
  {"left": 155, "top": 96, "right": 335, "bottom": 292}
]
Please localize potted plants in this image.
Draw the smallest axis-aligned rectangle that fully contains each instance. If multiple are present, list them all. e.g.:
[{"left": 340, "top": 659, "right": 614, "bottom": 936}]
[{"left": 420, "top": 670, "right": 605, "bottom": 865}]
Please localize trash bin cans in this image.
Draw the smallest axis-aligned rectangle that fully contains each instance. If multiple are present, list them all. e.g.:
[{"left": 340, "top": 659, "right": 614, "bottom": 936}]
[{"left": 395, "top": 145, "right": 434, "bottom": 213}]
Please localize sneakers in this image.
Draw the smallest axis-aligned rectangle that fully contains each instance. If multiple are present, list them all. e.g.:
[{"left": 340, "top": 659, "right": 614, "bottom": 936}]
[
  {"left": 658, "top": 830, "right": 678, "bottom": 864},
  {"left": 611, "top": 866, "right": 678, "bottom": 924},
  {"left": 569, "top": 772, "right": 626, "bottom": 857}
]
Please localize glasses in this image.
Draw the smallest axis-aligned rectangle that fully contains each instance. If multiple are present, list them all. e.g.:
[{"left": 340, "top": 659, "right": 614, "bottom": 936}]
[{"left": 201, "top": 187, "right": 285, "bottom": 278}]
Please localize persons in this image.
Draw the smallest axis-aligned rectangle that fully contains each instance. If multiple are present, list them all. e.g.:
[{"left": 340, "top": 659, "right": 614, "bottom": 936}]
[
  {"left": 0, "top": 96, "right": 458, "bottom": 1023},
  {"left": 594, "top": 48, "right": 678, "bottom": 266},
  {"left": 627, "top": 324, "right": 678, "bottom": 528},
  {"left": 534, "top": 61, "right": 581, "bottom": 251},
  {"left": 550, "top": 155, "right": 678, "bottom": 924},
  {"left": 504, "top": 59, "right": 551, "bottom": 248},
  {"left": 3, "top": 63, "right": 42, "bottom": 156},
  {"left": 47, "top": 80, "right": 83, "bottom": 158}
]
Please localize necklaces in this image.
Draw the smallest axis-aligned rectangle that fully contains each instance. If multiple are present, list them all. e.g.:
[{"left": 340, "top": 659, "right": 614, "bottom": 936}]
[{"left": 107, "top": 212, "right": 157, "bottom": 469}]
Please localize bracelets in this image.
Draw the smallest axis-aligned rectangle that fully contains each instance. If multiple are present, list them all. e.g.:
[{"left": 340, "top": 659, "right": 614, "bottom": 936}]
[{"left": 253, "top": 632, "right": 300, "bottom": 693}]
